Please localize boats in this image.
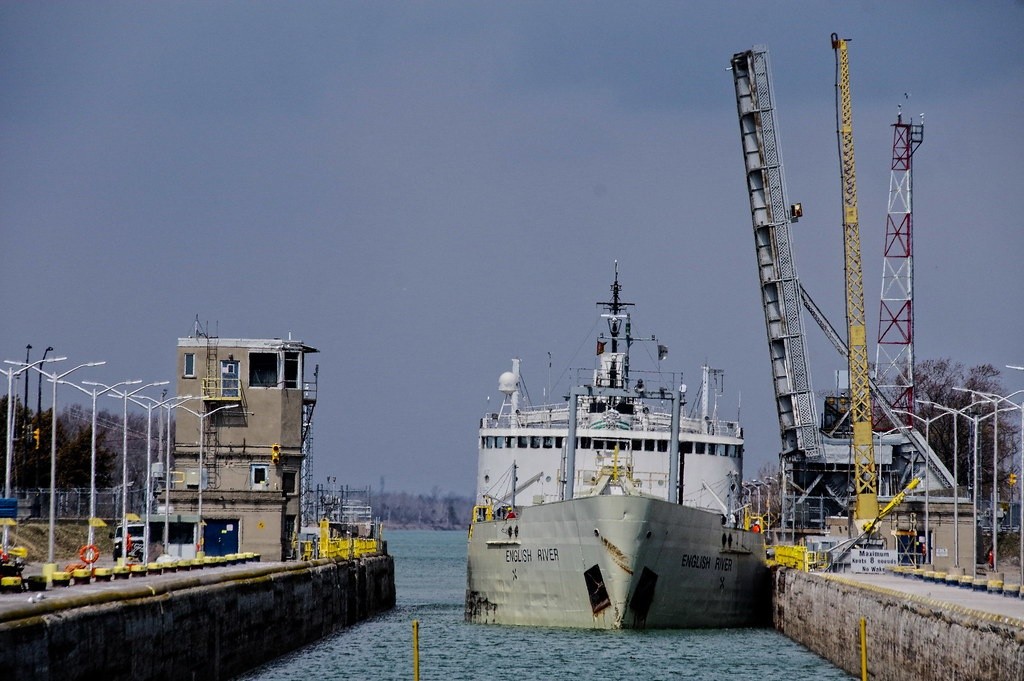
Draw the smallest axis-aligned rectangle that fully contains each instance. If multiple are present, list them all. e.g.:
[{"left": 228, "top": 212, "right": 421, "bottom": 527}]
[{"left": 462, "top": 259, "right": 773, "bottom": 629}]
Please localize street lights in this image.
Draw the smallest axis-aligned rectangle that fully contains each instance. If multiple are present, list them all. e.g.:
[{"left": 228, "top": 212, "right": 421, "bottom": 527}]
[
  {"left": 849, "top": 362, "right": 1024, "bottom": 569},
  {"left": 0, "top": 354, "right": 240, "bottom": 589}
]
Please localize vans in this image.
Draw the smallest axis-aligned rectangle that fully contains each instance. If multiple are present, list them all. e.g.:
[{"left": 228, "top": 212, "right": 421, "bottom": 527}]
[{"left": 109, "top": 522, "right": 145, "bottom": 561}]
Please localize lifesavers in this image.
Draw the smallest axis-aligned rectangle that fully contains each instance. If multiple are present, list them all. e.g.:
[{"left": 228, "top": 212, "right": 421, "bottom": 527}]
[
  {"left": 79, "top": 545, "right": 99, "bottom": 565},
  {"left": 989, "top": 551, "right": 993, "bottom": 567},
  {"left": 922, "top": 543, "right": 926, "bottom": 555}
]
